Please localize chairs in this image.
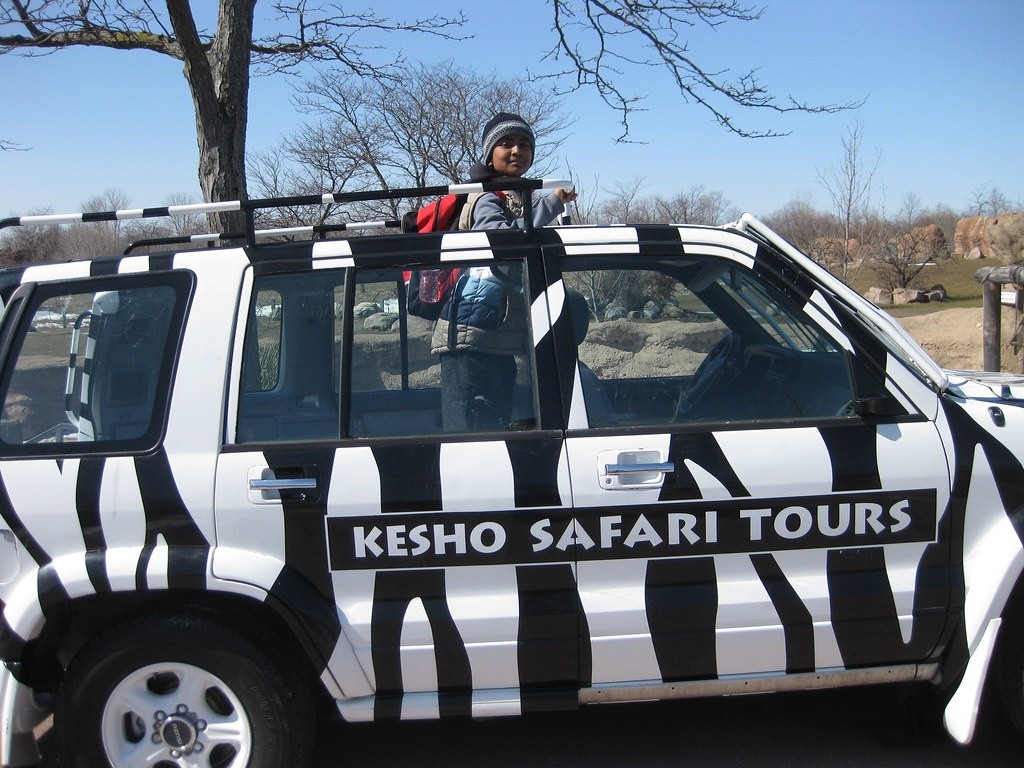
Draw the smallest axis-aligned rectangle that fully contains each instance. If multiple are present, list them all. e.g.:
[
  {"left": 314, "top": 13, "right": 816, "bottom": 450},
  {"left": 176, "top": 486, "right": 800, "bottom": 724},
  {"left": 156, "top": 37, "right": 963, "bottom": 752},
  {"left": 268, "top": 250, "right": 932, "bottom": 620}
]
[{"left": 565, "top": 285, "right": 619, "bottom": 427}]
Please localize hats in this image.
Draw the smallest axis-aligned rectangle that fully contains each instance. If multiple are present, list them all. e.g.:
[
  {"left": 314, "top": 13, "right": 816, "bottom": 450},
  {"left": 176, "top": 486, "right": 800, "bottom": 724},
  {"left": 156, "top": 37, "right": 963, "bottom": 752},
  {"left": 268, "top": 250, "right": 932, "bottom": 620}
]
[{"left": 481, "top": 111, "right": 534, "bottom": 170}]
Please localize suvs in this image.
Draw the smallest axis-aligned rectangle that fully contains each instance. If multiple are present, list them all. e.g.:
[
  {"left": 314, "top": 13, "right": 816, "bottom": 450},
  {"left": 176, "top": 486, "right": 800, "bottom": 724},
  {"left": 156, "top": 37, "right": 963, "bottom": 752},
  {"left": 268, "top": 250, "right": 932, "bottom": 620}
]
[{"left": 0, "top": 177, "right": 1024, "bottom": 768}]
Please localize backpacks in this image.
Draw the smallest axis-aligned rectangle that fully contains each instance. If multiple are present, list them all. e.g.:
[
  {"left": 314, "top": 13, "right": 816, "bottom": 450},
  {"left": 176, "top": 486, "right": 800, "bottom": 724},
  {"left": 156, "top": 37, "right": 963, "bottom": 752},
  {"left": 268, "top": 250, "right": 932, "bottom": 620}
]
[{"left": 400, "top": 190, "right": 506, "bottom": 322}]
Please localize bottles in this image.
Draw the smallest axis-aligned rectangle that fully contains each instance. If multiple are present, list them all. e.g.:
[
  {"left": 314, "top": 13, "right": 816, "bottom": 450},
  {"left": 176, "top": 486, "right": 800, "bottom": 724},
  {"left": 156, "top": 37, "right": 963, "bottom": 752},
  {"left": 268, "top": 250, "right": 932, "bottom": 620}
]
[{"left": 419, "top": 269, "right": 442, "bottom": 303}]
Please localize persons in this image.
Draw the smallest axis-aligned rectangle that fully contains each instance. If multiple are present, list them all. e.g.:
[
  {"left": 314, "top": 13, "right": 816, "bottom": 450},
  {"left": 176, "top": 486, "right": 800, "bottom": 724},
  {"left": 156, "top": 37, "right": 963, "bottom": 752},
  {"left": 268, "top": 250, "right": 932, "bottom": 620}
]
[{"left": 430, "top": 112, "right": 579, "bottom": 432}]
